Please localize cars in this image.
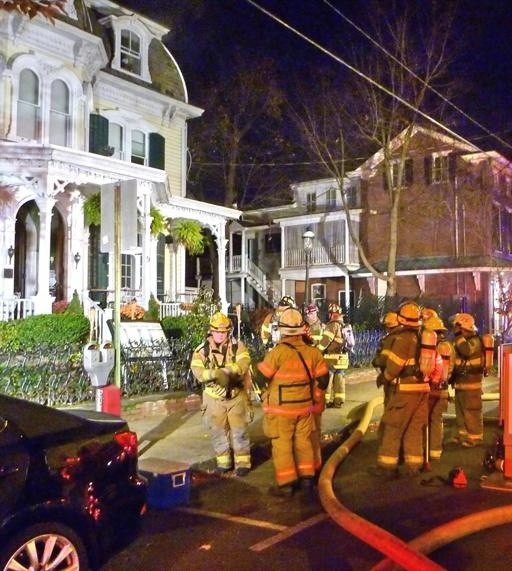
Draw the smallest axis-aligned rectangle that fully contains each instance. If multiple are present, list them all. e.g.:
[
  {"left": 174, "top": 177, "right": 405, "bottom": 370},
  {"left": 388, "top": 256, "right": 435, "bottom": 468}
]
[{"left": 1, "top": 393, "right": 148, "bottom": 571}]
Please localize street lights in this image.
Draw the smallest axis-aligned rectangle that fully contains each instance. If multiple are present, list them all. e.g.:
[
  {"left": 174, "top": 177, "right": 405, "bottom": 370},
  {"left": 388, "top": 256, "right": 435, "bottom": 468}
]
[{"left": 301, "top": 224, "right": 314, "bottom": 311}]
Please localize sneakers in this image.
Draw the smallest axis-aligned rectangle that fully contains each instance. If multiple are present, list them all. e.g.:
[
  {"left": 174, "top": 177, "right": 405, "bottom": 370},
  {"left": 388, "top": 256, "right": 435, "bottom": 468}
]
[
  {"left": 214, "top": 466, "right": 228, "bottom": 473},
  {"left": 376, "top": 466, "right": 420, "bottom": 476},
  {"left": 452, "top": 436, "right": 482, "bottom": 447},
  {"left": 236, "top": 467, "right": 249, "bottom": 476}
]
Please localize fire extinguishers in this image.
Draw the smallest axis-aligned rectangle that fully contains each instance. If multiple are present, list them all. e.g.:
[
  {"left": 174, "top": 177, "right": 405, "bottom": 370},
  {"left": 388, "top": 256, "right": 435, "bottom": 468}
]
[
  {"left": 483, "top": 329, "right": 494, "bottom": 376},
  {"left": 436, "top": 337, "right": 451, "bottom": 390},
  {"left": 419, "top": 329, "right": 437, "bottom": 382}
]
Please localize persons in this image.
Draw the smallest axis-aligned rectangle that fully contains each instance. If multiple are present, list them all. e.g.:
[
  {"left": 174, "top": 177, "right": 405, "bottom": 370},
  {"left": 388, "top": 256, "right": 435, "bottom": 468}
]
[
  {"left": 373, "top": 303, "right": 484, "bottom": 477},
  {"left": 256, "top": 297, "right": 349, "bottom": 498},
  {"left": 189, "top": 312, "right": 252, "bottom": 475}
]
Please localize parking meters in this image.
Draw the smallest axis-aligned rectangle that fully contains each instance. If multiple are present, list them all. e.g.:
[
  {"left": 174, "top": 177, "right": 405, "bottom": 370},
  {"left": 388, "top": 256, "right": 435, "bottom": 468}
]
[{"left": 83, "top": 340, "right": 116, "bottom": 414}]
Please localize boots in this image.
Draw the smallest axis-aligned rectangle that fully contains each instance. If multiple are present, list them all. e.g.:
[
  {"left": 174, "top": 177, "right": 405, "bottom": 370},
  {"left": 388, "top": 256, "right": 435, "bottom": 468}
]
[
  {"left": 270, "top": 482, "right": 294, "bottom": 497},
  {"left": 302, "top": 478, "right": 314, "bottom": 502}
]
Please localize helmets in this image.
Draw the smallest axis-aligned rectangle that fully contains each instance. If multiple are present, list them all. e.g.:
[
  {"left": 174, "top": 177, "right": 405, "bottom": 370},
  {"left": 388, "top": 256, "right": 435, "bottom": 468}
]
[
  {"left": 453, "top": 314, "right": 479, "bottom": 332},
  {"left": 277, "top": 309, "right": 306, "bottom": 336},
  {"left": 327, "top": 303, "right": 342, "bottom": 314},
  {"left": 210, "top": 312, "right": 232, "bottom": 332},
  {"left": 380, "top": 300, "right": 449, "bottom": 332},
  {"left": 279, "top": 294, "right": 298, "bottom": 309},
  {"left": 304, "top": 304, "right": 319, "bottom": 315}
]
